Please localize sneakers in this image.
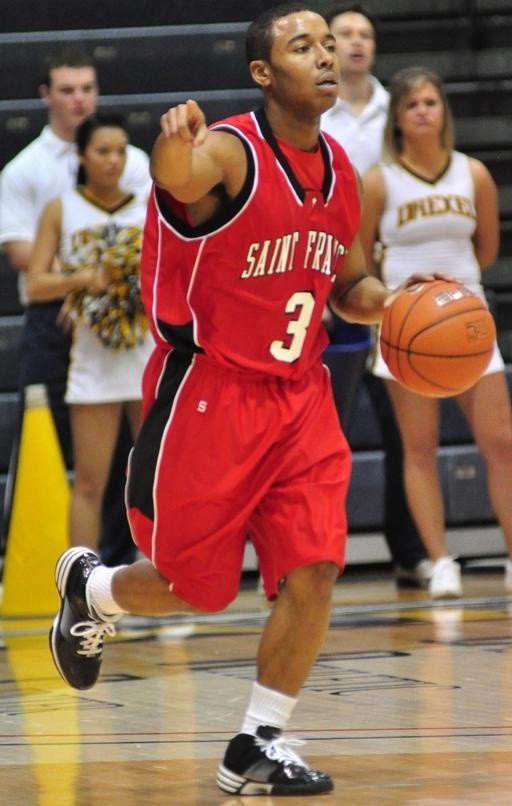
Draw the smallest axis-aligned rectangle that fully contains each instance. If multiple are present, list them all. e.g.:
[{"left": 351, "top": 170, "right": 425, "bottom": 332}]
[
  {"left": 49, "top": 546, "right": 122, "bottom": 691},
  {"left": 394, "top": 555, "right": 461, "bottom": 600},
  {"left": 216, "top": 725, "right": 333, "bottom": 795},
  {"left": 504, "top": 559, "right": 512, "bottom": 594}
]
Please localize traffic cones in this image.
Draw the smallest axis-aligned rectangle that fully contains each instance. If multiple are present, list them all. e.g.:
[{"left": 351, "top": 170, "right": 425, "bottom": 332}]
[
  {"left": 0, "top": 382, "right": 93, "bottom": 626},
  {"left": 0, "top": 626, "right": 80, "bottom": 806}
]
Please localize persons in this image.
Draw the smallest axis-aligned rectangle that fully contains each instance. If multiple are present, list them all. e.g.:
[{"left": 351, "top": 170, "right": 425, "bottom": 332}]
[
  {"left": 49, "top": 2, "right": 464, "bottom": 797},
  {"left": 360, "top": 68, "right": 512, "bottom": 598},
  {"left": 319, "top": 11, "right": 431, "bottom": 589}
]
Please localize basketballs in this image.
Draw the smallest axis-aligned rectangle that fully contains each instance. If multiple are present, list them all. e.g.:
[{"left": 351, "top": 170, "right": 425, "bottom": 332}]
[{"left": 381, "top": 282, "right": 498, "bottom": 398}]
